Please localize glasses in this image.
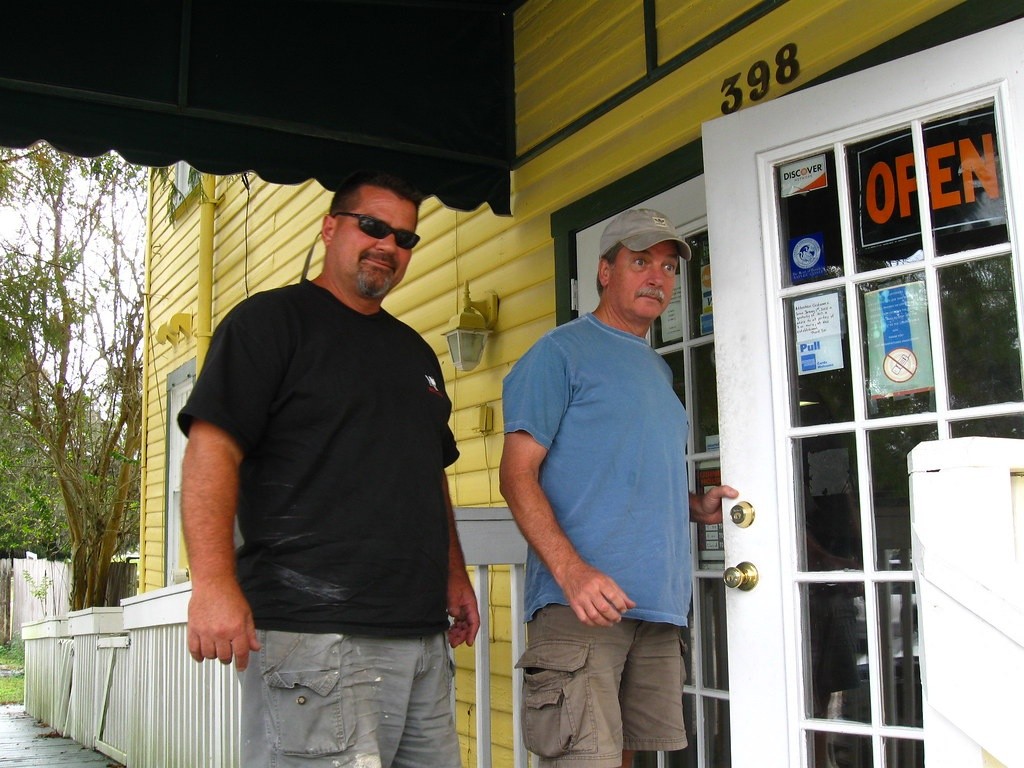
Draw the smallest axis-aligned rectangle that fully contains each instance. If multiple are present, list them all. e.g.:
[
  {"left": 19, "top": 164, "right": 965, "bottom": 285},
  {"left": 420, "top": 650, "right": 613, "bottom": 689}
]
[{"left": 333, "top": 211, "right": 420, "bottom": 249}]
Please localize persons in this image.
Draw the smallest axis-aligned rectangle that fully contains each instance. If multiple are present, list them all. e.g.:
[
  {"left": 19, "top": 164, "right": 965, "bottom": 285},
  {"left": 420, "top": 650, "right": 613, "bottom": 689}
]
[
  {"left": 801, "top": 442, "right": 864, "bottom": 768},
  {"left": 497, "top": 208, "right": 734, "bottom": 767},
  {"left": 177, "top": 169, "right": 479, "bottom": 767}
]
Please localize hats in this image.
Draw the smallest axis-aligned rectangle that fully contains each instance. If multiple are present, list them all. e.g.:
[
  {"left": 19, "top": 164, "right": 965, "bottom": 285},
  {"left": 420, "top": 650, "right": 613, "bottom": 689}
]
[{"left": 599, "top": 209, "right": 692, "bottom": 262}]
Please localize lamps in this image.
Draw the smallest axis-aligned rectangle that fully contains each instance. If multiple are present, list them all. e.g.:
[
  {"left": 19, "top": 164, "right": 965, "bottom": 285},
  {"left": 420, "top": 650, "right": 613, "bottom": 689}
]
[{"left": 440, "top": 277, "right": 499, "bottom": 373}]
